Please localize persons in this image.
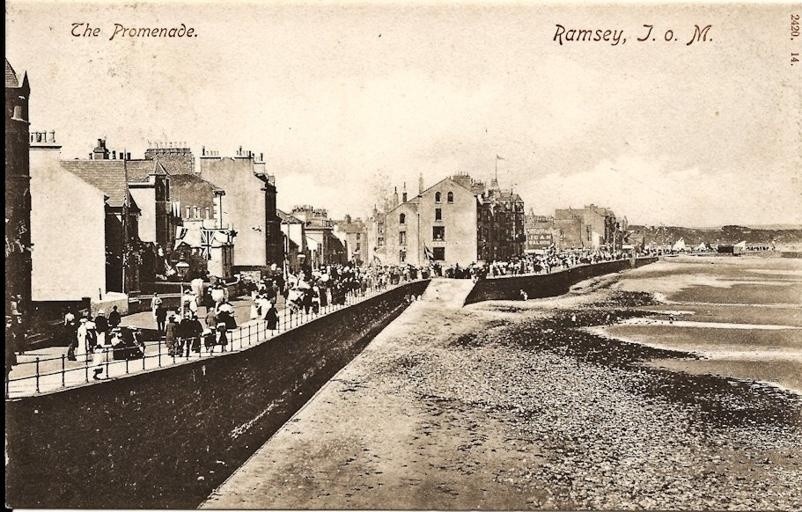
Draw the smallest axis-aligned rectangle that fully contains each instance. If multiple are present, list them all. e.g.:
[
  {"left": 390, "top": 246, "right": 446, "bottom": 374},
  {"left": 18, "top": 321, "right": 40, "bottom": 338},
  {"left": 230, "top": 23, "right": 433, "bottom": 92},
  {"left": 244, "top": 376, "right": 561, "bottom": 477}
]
[{"left": 65, "top": 248, "right": 678, "bottom": 380}]
